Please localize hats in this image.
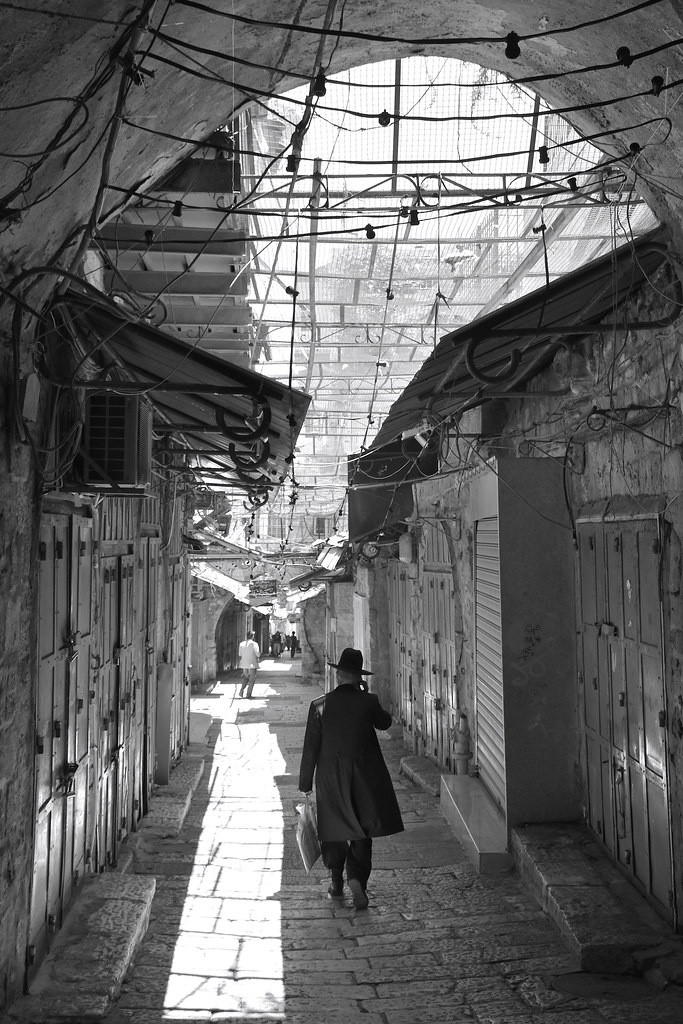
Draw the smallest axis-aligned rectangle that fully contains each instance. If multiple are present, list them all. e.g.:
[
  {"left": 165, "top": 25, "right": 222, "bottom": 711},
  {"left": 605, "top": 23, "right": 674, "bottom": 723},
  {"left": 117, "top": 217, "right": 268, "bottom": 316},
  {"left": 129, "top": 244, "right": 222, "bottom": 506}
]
[{"left": 326, "top": 647, "right": 373, "bottom": 675}]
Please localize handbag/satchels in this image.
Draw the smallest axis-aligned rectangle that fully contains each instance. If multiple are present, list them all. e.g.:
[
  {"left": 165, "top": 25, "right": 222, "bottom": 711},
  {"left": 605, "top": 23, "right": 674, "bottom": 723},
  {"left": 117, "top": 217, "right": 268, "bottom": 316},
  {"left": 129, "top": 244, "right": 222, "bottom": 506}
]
[{"left": 295, "top": 794, "right": 321, "bottom": 873}]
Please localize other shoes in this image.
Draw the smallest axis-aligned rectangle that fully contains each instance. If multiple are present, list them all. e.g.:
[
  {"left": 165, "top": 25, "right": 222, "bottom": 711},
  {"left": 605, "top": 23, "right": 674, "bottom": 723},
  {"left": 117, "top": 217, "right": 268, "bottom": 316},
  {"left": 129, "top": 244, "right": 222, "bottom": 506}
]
[
  {"left": 347, "top": 877, "right": 369, "bottom": 909},
  {"left": 327, "top": 882, "right": 343, "bottom": 896}
]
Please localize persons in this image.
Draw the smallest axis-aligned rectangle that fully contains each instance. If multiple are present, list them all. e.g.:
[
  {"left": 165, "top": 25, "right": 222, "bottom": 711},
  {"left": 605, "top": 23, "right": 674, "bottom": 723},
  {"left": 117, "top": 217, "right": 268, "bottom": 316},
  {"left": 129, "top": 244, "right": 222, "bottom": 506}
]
[
  {"left": 298, "top": 646, "right": 406, "bottom": 910},
  {"left": 270, "top": 630, "right": 298, "bottom": 659},
  {"left": 238, "top": 630, "right": 260, "bottom": 699}
]
